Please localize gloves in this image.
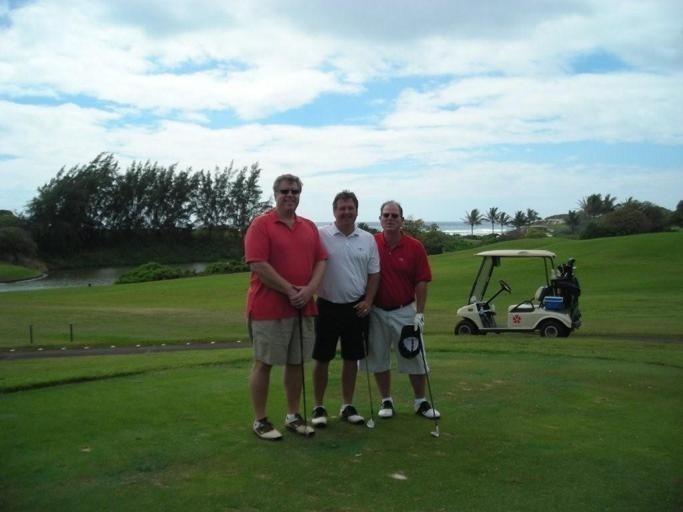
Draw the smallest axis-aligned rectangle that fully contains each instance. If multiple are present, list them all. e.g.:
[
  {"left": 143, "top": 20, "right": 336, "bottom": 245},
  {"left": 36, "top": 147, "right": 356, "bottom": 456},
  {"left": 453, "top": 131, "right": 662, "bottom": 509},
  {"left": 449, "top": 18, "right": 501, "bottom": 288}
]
[{"left": 414, "top": 313, "right": 425, "bottom": 330}]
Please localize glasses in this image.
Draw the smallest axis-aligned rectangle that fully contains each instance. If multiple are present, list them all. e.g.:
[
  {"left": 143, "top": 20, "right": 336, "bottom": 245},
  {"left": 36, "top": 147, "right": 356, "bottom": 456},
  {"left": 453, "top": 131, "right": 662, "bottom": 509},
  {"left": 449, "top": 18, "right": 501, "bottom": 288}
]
[
  {"left": 381, "top": 212, "right": 400, "bottom": 218},
  {"left": 277, "top": 189, "right": 300, "bottom": 195}
]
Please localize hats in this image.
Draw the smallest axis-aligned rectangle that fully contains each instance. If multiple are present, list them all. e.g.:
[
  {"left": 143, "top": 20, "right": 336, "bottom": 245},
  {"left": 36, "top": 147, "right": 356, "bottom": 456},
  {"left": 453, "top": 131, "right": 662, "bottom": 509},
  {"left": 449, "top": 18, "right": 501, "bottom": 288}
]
[{"left": 398, "top": 325, "right": 421, "bottom": 358}]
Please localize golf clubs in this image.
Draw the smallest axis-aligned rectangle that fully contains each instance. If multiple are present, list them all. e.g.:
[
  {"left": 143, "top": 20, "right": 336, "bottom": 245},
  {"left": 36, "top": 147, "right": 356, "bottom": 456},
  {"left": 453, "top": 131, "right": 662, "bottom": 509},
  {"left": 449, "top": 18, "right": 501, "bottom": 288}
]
[
  {"left": 299, "top": 302, "right": 309, "bottom": 437},
  {"left": 418, "top": 328, "right": 440, "bottom": 438},
  {"left": 363, "top": 316, "right": 375, "bottom": 429}
]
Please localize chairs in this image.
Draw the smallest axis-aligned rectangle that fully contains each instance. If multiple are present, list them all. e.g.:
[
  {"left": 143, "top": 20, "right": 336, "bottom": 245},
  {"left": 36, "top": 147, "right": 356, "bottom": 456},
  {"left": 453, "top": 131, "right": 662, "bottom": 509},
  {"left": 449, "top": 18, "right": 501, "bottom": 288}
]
[{"left": 505, "top": 286, "right": 550, "bottom": 313}]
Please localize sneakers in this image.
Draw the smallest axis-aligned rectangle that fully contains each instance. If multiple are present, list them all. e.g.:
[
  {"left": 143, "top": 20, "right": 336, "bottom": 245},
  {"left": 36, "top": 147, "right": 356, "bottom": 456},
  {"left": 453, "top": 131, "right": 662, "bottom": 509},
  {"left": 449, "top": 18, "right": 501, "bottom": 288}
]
[
  {"left": 340, "top": 405, "right": 364, "bottom": 423},
  {"left": 378, "top": 400, "right": 395, "bottom": 417},
  {"left": 311, "top": 406, "right": 327, "bottom": 424},
  {"left": 284, "top": 414, "right": 314, "bottom": 436},
  {"left": 254, "top": 418, "right": 282, "bottom": 440},
  {"left": 414, "top": 401, "right": 441, "bottom": 419}
]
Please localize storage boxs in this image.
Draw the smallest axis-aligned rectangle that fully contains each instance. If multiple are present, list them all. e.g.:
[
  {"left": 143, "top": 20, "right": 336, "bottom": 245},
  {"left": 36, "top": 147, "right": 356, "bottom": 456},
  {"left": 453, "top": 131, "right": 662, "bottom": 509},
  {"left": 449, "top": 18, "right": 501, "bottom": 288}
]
[{"left": 542, "top": 295, "right": 566, "bottom": 311}]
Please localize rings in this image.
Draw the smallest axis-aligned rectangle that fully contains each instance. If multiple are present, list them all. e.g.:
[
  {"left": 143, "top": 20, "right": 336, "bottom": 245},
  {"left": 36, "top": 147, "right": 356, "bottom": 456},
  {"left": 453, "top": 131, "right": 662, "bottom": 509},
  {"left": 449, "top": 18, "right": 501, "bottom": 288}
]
[
  {"left": 364, "top": 308, "right": 368, "bottom": 312},
  {"left": 300, "top": 300, "right": 304, "bottom": 305}
]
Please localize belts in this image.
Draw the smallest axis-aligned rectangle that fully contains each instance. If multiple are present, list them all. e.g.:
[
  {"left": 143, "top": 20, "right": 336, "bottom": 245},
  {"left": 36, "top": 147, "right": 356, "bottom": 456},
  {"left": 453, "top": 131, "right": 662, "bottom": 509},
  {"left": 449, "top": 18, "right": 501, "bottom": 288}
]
[{"left": 373, "top": 299, "right": 415, "bottom": 312}]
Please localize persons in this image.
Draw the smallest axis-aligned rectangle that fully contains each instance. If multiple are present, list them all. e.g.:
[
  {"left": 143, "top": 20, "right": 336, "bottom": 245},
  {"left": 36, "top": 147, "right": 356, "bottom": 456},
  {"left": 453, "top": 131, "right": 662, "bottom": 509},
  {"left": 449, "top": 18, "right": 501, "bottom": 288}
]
[
  {"left": 244, "top": 175, "right": 327, "bottom": 442},
  {"left": 311, "top": 191, "right": 381, "bottom": 428},
  {"left": 357, "top": 201, "right": 442, "bottom": 420}
]
